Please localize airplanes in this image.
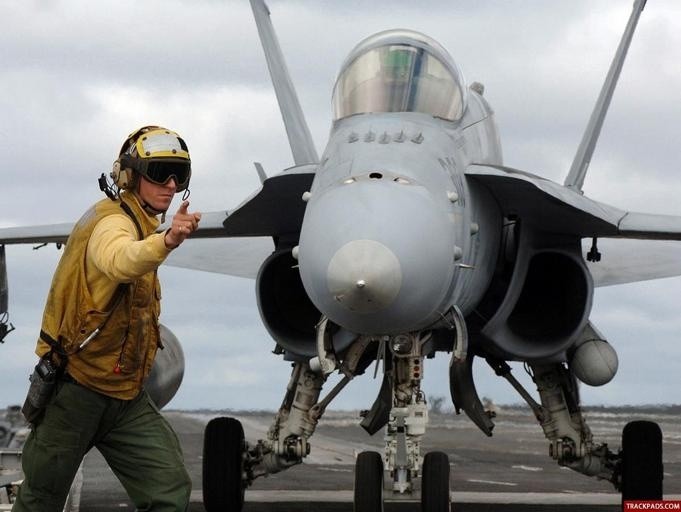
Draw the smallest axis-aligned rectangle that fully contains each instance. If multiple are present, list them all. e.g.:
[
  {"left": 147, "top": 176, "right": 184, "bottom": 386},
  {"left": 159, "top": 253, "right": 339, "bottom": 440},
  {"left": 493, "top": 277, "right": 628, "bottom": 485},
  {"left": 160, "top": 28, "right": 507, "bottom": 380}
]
[{"left": 1, "top": 0, "right": 679, "bottom": 510}]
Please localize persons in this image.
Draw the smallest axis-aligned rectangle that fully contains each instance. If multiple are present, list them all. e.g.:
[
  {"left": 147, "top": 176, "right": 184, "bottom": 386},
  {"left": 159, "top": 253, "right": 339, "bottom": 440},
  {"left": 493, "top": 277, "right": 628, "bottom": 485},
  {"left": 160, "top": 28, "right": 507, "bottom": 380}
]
[{"left": 10, "top": 122, "right": 205, "bottom": 510}]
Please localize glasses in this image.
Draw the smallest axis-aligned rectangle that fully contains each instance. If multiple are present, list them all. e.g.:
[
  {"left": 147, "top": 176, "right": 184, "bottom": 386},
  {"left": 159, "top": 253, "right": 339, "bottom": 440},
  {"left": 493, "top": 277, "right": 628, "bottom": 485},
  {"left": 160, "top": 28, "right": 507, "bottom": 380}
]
[{"left": 121, "top": 157, "right": 191, "bottom": 186}]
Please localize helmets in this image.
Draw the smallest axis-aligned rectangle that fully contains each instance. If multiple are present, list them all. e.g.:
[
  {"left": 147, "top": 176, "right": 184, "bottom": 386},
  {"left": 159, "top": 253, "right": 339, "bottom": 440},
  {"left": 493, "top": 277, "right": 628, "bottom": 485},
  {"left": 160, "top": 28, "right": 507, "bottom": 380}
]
[{"left": 111, "top": 125, "right": 192, "bottom": 193}]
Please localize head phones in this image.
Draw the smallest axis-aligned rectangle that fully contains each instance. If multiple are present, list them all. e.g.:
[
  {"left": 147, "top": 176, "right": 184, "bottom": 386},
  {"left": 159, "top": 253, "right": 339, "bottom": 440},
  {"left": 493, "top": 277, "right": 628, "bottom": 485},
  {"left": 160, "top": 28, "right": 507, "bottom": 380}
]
[{"left": 110, "top": 139, "right": 139, "bottom": 193}]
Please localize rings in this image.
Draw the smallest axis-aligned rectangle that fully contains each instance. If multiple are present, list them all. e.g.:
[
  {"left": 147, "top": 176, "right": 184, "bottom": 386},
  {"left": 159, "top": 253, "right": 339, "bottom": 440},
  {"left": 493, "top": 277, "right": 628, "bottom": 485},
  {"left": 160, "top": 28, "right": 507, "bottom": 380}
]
[{"left": 178, "top": 225, "right": 182, "bottom": 232}]
